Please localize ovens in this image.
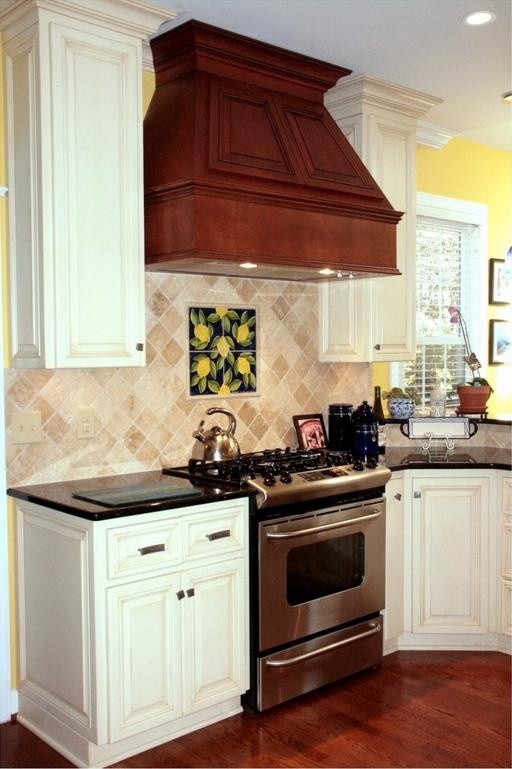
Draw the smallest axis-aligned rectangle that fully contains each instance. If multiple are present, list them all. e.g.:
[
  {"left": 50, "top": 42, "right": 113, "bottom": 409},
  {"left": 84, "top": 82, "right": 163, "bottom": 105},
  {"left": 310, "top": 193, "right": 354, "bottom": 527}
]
[{"left": 255, "top": 496, "right": 386, "bottom": 714}]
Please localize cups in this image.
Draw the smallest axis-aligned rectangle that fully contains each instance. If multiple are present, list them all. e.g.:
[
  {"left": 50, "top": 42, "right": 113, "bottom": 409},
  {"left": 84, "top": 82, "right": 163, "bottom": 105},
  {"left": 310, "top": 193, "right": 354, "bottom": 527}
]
[{"left": 429, "top": 390, "right": 446, "bottom": 418}]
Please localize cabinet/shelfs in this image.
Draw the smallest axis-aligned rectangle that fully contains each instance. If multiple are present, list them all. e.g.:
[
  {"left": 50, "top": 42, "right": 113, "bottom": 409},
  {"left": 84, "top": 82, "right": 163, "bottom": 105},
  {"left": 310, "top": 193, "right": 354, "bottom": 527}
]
[
  {"left": 383, "top": 470, "right": 511, "bottom": 658},
  {"left": 2, "top": 0, "right": 176, "bottom": 368},
  {"left": 12, "top": 498, "right": 249, "bottom": 768},
  {"left": 316, "top": 74, "right": 446, "bottom": 366}
]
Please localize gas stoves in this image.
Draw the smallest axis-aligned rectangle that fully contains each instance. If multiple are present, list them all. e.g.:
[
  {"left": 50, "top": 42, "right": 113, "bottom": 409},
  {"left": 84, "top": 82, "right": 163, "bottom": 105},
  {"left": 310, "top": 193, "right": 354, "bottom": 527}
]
[{"left": 161, "top": 447, "right": 392, "bottom": 510}]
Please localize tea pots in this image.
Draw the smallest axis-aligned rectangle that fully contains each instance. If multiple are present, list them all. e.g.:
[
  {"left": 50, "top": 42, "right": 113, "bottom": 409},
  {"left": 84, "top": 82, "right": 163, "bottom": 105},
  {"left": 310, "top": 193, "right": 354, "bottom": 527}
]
[{"left": 191, "top": 406, "right": 242, "bottom": 463}]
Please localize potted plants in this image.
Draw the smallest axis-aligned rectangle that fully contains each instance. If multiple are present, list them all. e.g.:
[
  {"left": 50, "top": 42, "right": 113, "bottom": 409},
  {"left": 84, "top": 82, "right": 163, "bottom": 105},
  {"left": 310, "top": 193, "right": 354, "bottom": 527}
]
[{"left": 383, "top": 376, "right": 494, "bottom": 419}]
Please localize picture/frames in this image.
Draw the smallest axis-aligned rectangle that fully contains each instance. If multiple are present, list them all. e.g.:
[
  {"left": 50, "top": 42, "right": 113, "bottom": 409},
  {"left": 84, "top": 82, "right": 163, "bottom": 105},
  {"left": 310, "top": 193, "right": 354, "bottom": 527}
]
[
  {"left": 490, "top": 257, "right": 512, "bottom": 365},
  {"left": 184, "top": 301, "right": 328, "bottom": 450}
]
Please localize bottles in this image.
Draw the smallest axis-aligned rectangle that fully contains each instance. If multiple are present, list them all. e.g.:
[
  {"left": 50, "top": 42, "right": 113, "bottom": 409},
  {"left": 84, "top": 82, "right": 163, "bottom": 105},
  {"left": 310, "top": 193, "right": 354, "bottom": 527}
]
[
  {"left": 326, "top": 402, "right": 353, "bottom": 458},
  {"left": 353, "top": 401, "right": 379, "bottom": 461},
  {"left": 371, "top": 385, "right": 387, "bottom": 456}
]
